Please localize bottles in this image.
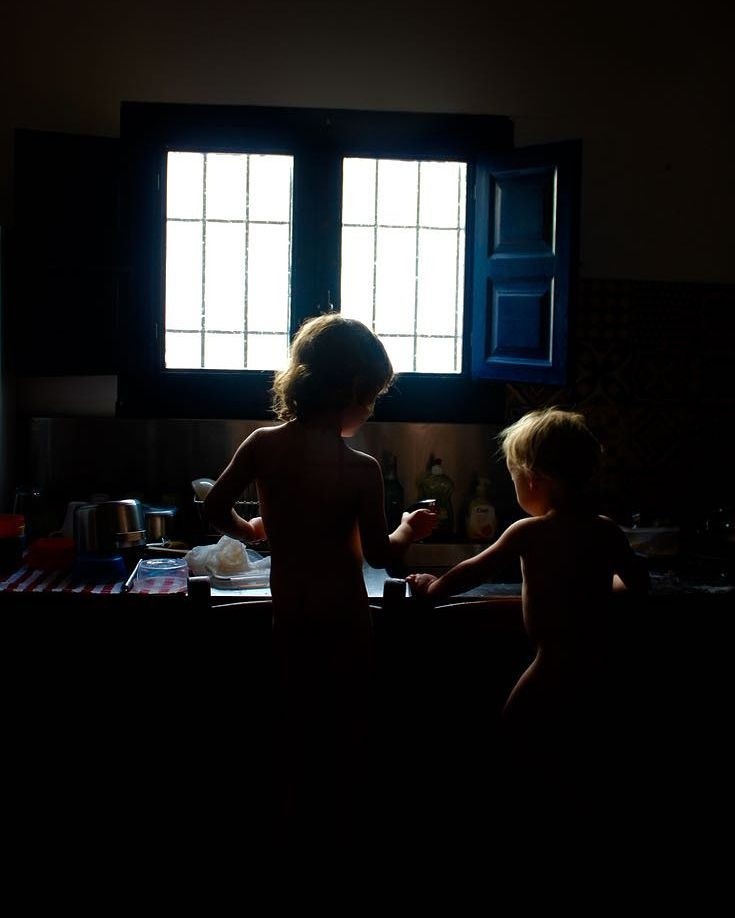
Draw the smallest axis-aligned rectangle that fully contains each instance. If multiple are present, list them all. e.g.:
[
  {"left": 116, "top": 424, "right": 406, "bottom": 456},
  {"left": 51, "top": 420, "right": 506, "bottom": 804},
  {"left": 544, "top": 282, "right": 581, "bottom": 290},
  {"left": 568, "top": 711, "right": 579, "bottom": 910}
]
[
  {"left": 418, "top": 458, "right": 455, "bottom": 545},
  {"left": 463, "top": 480, "right": 496, "bottom": 543},
  {"left": 383, "top": 456, "right": 404, "bottom": 531}
]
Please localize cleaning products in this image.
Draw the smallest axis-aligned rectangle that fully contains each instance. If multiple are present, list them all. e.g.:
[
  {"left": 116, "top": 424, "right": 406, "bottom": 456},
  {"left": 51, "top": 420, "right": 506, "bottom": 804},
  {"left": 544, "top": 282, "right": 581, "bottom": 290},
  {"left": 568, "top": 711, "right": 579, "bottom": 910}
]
[{"left": 423, "top": 459, "right": 455, "bottom": 538}]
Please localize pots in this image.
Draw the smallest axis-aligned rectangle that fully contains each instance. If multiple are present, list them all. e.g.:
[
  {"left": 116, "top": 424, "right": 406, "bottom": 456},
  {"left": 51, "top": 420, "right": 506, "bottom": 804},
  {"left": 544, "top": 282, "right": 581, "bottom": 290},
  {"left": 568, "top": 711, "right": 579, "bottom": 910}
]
[{"left": 73, "top": 498, "right": 147, "bottom": 551}]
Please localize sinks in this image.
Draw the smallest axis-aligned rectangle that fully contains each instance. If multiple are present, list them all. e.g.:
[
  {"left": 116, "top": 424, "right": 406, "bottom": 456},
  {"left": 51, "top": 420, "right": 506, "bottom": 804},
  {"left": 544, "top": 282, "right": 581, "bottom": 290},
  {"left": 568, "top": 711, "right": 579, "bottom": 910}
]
[{"left": 384, "top": 544, "right": 523, "bottom": 598}]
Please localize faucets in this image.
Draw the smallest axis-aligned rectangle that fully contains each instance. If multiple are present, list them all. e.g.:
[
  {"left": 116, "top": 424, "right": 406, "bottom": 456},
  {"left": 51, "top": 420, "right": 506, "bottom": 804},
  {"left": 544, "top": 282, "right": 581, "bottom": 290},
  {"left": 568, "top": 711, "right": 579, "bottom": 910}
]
[{"left": 404, "top": 499, "right": 440, "bottom": 544}]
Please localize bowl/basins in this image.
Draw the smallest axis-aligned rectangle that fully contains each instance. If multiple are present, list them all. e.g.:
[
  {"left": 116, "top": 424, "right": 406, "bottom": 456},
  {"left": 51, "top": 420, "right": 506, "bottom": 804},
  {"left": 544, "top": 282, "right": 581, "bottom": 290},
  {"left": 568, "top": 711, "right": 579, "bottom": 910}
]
[
  {"left": 191, "top": 478, "right": 216, "bottom": 501},
  {"left": 79, "top": 554, "right": 128, "bottom": 584},
  {"left": 134, "top": 558, "right": 188, "bottom": 592}
]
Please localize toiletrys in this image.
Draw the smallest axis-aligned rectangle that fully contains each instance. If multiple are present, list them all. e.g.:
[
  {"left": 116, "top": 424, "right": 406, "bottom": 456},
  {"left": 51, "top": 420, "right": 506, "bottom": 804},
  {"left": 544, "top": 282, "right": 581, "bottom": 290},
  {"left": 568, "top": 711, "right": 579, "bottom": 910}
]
[{"left": 465, "top": 477, "right": 499, "bottom": 542}]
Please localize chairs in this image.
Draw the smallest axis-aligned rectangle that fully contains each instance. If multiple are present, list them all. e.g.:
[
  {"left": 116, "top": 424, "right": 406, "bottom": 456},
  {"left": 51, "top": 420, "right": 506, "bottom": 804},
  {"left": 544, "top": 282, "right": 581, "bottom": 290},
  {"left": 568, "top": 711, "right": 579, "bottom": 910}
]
[{"left": 186, "top": 576, "right": 521, "bottom": 611}]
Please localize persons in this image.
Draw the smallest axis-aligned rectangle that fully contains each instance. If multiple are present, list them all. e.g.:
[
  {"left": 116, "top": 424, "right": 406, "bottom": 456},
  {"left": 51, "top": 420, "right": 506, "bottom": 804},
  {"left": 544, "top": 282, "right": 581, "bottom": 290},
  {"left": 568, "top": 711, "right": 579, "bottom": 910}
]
[
  {"left": 407, "top": 403, "right": 650, "bottom": 795},
  {"left": 201, "top": 313, "right": 438, "bottom": 833}
]
